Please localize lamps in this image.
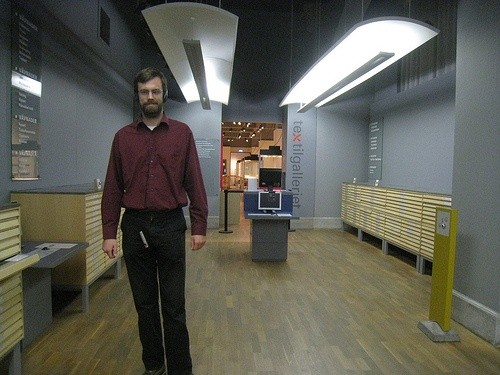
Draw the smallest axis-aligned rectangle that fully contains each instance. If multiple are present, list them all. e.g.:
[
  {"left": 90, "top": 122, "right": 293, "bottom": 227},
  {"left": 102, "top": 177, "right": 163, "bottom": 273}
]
[
  {"left": 141, "top": 0, "right": 240, "bottom": 111},
  {"left": 279, "top": 0, "right": 440, "bottom": 111}
]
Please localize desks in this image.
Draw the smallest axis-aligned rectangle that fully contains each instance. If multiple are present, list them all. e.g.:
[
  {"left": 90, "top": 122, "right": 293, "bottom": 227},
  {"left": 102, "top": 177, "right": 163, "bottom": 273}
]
[{"left": 21, "top": 238, "right": 91, "bottom": 349}]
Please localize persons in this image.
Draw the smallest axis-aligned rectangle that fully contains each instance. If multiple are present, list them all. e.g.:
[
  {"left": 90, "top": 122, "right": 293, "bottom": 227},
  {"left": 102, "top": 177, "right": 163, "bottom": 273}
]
[{"left": 101, "top": 68, "right": 209, "bottom": 375}]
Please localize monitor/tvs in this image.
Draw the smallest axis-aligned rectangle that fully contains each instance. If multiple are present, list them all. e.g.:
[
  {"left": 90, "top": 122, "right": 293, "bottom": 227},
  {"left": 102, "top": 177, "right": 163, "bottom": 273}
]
[
  {"left": 259, "top": 168, "right": 282, "bottom": 191},
  {"left": 258, "top": 191, "right": 281, "bottom": 213}
]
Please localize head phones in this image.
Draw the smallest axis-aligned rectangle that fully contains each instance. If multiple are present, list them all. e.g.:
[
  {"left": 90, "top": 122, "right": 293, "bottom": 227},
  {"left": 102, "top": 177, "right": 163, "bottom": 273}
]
[{"left": 160, "top": 71, "right": 168, "bottom": 103}]
